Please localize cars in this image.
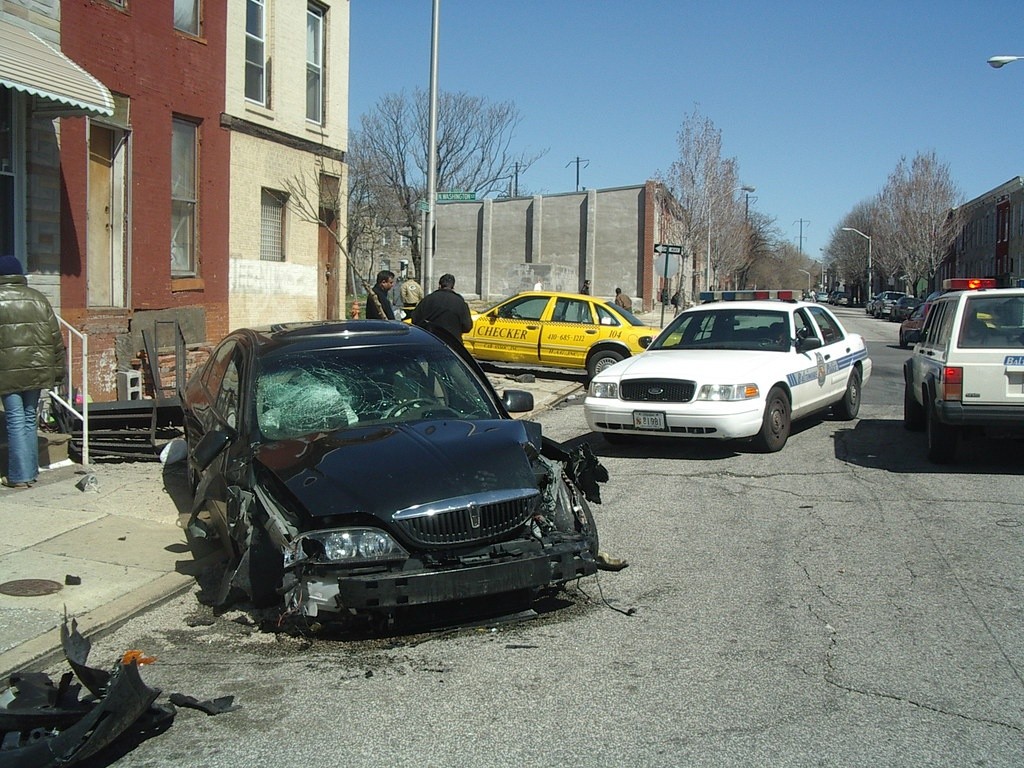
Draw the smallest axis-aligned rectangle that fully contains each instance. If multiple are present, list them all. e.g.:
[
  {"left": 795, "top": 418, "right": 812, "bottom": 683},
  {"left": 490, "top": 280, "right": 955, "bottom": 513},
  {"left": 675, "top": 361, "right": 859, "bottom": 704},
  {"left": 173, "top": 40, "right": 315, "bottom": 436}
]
[
  {"left": 815, "top": 292, "right": 829, "bottom": 302},
  {"left": 866, "top": 291, "right": 908, "bottom": 319},
  {"left": 890, "top": 296, "right": 922, "bottom": 322},
  {"left": 182, "top": 319, "right": 610, "bottom": 631},
  {"left": 827, "top": 290, "right": 853, "bottom": 307},
  {"left": 404, "top": 291, "right": 684, "bottom": 381},
  {"left": 583, "top": 300, "right": 872, "bottom": 452}
]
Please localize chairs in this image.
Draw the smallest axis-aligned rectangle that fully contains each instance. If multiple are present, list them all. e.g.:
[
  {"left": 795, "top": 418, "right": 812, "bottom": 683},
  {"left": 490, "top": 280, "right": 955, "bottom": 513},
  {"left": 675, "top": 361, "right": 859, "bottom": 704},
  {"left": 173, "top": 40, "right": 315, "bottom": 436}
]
[{"left": 771, "top": 322, "right": 797, "bottom": 339}]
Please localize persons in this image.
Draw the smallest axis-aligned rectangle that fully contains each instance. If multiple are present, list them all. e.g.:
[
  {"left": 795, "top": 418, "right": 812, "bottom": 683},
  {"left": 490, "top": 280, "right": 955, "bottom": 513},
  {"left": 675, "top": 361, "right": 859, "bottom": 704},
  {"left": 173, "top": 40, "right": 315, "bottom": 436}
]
[
  {"left": 411, "top": 274, "right": 473, "bottom": 345},
  {"left": 802, "top": 289, "right": 817, "bottom": 302},
  {"left": 671, "top": 292, "right": 679, "bottom": 305},
  {"left": 366, "top": 270, "right": 395, "bottom": 321},
  {"left": 400, "top": 273, "right": 424, "bottom": 318},
  {"left": 615, "top": 288, "right": 632, "bottom": 314},
  {"left": 0, "top": 256, "right": 66, "bottom": 487},
  {"left": 581, "top": 280, "right": 591, "bottom": 295}
]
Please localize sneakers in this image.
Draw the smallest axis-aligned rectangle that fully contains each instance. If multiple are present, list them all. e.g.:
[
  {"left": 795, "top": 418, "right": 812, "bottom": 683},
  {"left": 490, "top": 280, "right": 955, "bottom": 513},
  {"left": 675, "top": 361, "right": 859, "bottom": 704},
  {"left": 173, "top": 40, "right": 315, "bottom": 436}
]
[{"left": 2, "top": 474, "right": 34, "bottom": 489}]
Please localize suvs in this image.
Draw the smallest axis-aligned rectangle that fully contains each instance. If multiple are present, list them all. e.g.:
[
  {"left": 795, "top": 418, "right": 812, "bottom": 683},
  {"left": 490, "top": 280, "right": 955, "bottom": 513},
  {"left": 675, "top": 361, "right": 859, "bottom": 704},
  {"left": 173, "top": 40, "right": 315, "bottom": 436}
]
[{"left": 904, "top": 288, "right": 1024, "bottom": 463}]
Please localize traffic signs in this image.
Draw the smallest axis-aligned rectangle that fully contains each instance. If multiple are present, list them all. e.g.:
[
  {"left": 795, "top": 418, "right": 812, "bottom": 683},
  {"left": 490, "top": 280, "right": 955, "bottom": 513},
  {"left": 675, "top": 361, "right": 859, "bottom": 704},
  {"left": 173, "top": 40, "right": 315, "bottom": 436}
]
[
  {"left": 419, "top": 200, "right": 429, "bottom": 211},
  {"left": 653, "top": 244, "right": 681, "bottom": 254},
  {"left": 435, "top": 190, "right": 476, "bottom": 202}
]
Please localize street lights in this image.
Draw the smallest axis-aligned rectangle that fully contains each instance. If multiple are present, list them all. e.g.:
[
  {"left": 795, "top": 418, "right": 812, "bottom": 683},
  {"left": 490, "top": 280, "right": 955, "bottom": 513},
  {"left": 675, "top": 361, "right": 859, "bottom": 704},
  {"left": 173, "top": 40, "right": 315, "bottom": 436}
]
[
  {"left": 706, "top": 185, "right": 755, "bottom": 292},
  {"left": 842, "top": 227, "right": 871, "bottom": 300}
]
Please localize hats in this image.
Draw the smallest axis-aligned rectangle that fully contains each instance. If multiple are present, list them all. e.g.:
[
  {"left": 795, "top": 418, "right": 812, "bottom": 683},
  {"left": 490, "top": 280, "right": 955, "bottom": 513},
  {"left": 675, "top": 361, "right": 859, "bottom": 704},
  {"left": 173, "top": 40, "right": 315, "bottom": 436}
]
[{"left": 0, "top": 255, "right": 24, "bottom": 275}]
